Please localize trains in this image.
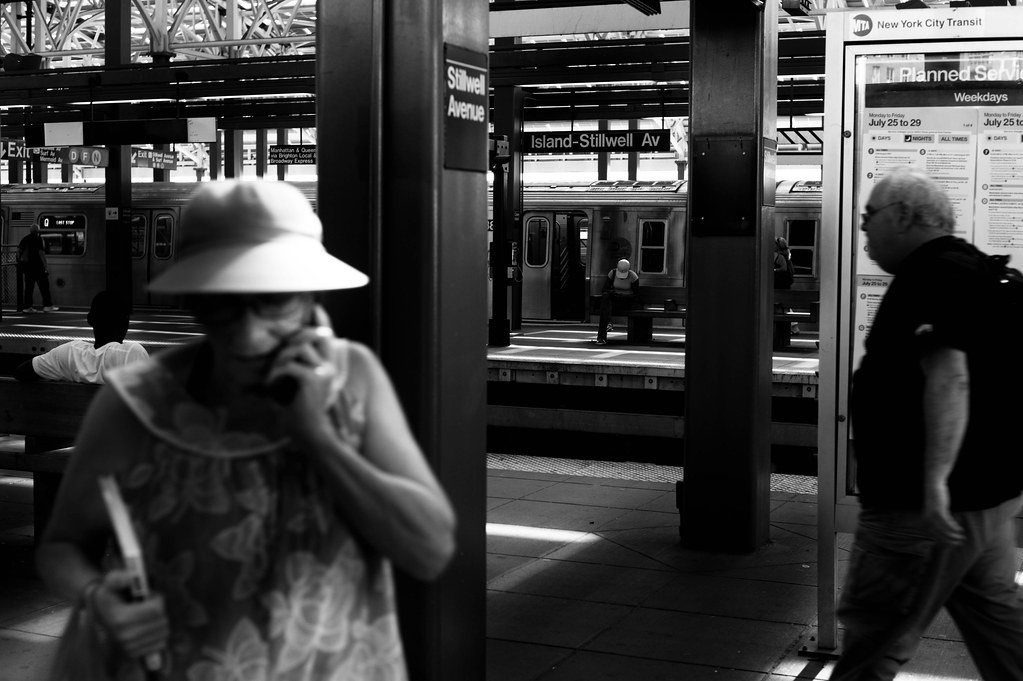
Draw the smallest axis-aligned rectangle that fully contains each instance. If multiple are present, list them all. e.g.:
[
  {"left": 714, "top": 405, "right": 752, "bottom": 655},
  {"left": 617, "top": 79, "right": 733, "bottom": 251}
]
[{"left": 0, "top": 177, "right": 820, "bottom": 336}]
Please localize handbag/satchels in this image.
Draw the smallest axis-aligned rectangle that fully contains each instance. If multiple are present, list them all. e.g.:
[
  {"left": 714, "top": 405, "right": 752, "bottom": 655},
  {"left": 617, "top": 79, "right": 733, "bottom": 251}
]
[{"left": 49, "top": 578, "right": 170, "bottom": 680}]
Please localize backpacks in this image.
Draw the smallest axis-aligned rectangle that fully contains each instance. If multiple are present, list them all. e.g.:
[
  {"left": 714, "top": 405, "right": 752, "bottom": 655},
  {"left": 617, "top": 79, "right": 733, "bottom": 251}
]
[
  {"left": 973, "top": 252, "right": 1023, "bottom": 476},
  {"left": 18, "top": 244, "right": 29, "bottom": 272}
]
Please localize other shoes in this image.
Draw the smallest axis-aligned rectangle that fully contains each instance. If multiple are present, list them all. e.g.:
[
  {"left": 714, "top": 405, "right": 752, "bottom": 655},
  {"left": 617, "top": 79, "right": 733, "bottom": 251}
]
[
  {"left": 595, "top": 338, "right": 606, "bottom": 344},
  {"left": 23, "top": 306, "right": 37, "bottom": 313},
  {"left": 43, "top": 305, "right": 59, "bottom": 311}
]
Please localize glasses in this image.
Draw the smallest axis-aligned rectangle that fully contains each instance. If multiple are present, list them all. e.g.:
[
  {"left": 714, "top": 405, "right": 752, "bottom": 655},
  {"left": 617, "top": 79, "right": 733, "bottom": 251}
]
[
  {"left": 863, "top": 199, "right": 903, "bottom": 221},
  {"left": 187, "top": 292, "right": 303, "bottom": 319}
]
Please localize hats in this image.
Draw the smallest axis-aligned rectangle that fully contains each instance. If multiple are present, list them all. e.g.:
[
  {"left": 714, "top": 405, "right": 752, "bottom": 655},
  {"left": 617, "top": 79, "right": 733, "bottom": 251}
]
[
  {"left": 144, "top": 177, "right": 375, "bottom": 298},
  {"left": 30, "top": 223, "right": 40, "bottom": 232},
  {"left": 616, "top": 258, "right": 631, "bottom": 279}
]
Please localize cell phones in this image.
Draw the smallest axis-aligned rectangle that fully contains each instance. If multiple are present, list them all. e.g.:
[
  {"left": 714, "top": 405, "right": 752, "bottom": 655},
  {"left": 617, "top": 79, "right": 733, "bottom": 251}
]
[{"left": 264, "top": 319, "right": 317, "bottom": 418}]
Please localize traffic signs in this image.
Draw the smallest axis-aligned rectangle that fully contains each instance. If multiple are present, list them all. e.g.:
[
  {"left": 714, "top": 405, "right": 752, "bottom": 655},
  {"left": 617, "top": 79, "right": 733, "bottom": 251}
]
[
  {"left": 1, "top": 132, "right": 178, "bottom": 171},
  {"left": 520, "top": 128, "right": 671, "bottom": 154}
]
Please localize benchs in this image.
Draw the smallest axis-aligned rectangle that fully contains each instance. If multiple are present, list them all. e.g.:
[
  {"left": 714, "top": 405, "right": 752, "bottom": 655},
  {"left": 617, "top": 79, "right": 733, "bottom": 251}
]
[
  {"left": 28, "top": 375, "right": 109, "bottom": 552},
  {"left": 592, "top": 304, "right": 687, "bottom": 343},
  {"left": 769, "top": 311, "right": 812, "bottom": 348}
]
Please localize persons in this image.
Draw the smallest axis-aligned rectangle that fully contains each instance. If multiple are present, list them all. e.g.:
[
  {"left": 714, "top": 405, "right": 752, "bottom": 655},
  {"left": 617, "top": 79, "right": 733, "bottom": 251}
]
[
  {"left": 776, "top": 236, "right": 800, "bottom": 336},
  {"left": 34, "top": 176, "right": 462, "bottom": 681},
  {"left": 594, "top": 259, "right": 643, "bottom": 346},
  {"left": 812, "top": 168, "right": 1023, "bottom": 681},
  {"left": 31, "top": 290, "right": 151, "bottom": 387},
  {"left": 15, "top": 223, "right": 61, "bottom": 314},
  {"left": 773, "top": 250, "right": 788, "bottom": 290}
]
